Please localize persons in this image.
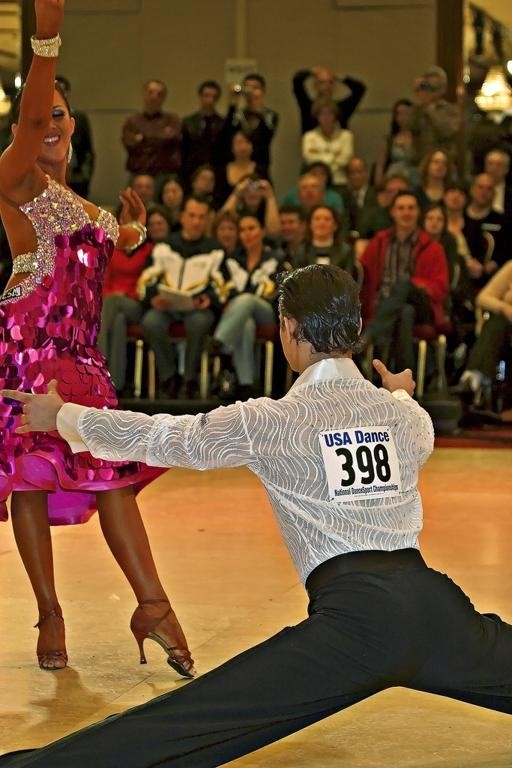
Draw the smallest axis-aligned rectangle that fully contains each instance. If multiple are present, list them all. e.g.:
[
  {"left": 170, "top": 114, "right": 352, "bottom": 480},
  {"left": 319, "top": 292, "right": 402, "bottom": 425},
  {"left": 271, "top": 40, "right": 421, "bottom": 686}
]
[
  {"left": 412, "top": 66, "right": 458, "bottom": 170},
  {"left": 214, "top": 214, "right": 289, "bottom": 398},
  {"left": 463, "top": 176, "right": 507, "bottom": 258},
  {"left": 122, "top": 81, "right": 183, "bottom": 196},
  {"left": 344, "top": 159, "right": 368, "bottom": 190},
  {"left": 375, "top": 99, "right": 412, "bottom": 185},
  {"left": 0, "top": 0, "right": 197, "bottom": 681},
  {"left": 484, "top": 150, "right": 508, "bottom": 179},
  {"left": 100, "top": 193, "right": 152, "bottom": 397},
  {"left": 419, "top": 206, "right": 448, "bottom": 236},
  {"left": 416, "top": 150, "right": 452, "bottom": 220},
  {"left": 274, "top": 210, "right": 298, "bottom": 244},
  {"left": 1, "top": 263, "right": 512, "bottom": 767},
  {"left": 300, "top": 97, "right": 354, "bottom": 189},
  {"left": 188, "top": 164, "right": 217, "bottom": 197},
  {"left": 156, "top": 176, "right": 186, "bottom": 216},
  {"left": 364, "top": 174, "right": 405, "bottom": 243},
  {"left": 214, "top": 74, "right": 280, "bottom": 206},
  {"left": 215, "top": 214, "right": 237, "bottom": 249},
  {"left": 144, "top": 211, "right": 171, "bottom": 241},
  {"left": 217, "top": 132, "right": 269, "bottom": 202},
  {"left": 296, "top": 174, "right": 324, "bottom": 218},
  {"left": 291, "top": 210, "right": 350, "bottom": 266},
  {"left": 137, "top": 200, "right": 225, "bottom": 396},
  {"left": 283, "top": 164, "right": 347, "bottom": 218},
  {"left": 128, "top": 172, "right": 158, "bottom": 206},
  {"left": 443, "top": 182, "right": 482, "bottom": 263},
  {"left": 182, "top": 82, "right": 227, "bottom": 195},
  {"left": 358, "top": 193, "right": 448, "bottom": 378},
  {"left": 461, "top": 258, "right": 511, "bottom": 403},
  {"left": 291, "top": 66, "right": 366, "bottom": 174},
  {"left": 53, "top": 71, "right": 97, "bottom": 199}
]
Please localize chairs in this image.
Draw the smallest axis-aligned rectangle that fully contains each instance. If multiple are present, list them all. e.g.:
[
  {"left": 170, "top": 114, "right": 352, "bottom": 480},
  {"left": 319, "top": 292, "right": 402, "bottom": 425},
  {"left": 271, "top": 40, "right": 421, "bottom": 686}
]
[{"left": 124, "top": 233, "right": 493, "bottom": 402}]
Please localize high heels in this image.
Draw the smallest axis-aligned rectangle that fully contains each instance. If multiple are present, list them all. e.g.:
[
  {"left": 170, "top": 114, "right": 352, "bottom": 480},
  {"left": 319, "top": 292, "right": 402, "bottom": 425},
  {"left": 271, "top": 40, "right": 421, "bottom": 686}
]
[
  {"left": 33, "top": 605, "right": 68, "bottom": 671},
  {"left": 130, "top": 595, "right": 197, "bottom": 678}
]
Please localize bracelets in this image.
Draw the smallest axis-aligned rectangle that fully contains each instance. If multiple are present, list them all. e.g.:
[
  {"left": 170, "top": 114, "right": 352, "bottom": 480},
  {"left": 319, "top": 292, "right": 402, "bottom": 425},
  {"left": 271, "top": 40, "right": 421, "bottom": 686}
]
[{"left": 31, "top": 32, "right": 60, "bottom": 58}]
